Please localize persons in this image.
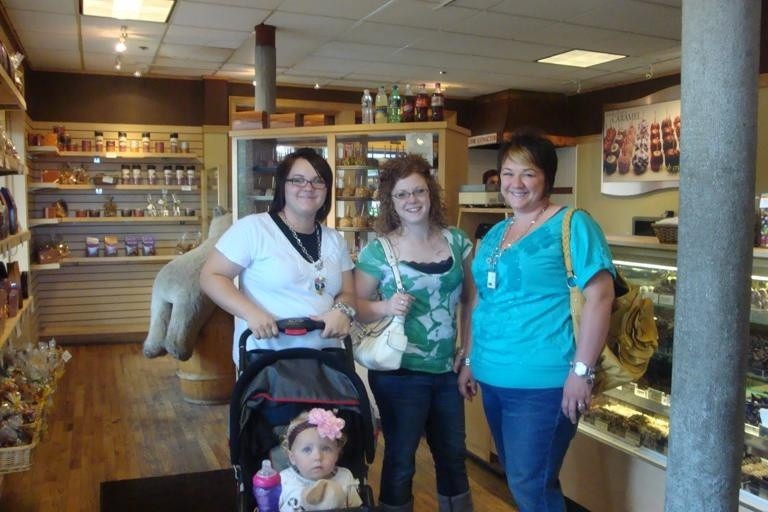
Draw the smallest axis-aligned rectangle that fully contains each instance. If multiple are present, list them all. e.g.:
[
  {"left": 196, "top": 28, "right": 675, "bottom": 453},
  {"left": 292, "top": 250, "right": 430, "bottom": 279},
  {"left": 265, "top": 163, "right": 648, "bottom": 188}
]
[
  {"left": 199, "top": 147, "right": 357, "bottom": 381},
  {"left": 352, "top": 153, "right": 475, "bottom": 512},
  {"left": 277, "top": 411, "right": 362, "bottom": 512},
  {"left": 456, "top": 132, "right": 618, "bottom": 512}
]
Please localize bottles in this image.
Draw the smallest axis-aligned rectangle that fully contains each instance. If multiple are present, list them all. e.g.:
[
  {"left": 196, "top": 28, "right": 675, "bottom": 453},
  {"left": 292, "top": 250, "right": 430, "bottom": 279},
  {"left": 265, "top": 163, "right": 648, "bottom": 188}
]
[
  {"left": 155, "top": 141, "right": 164, "bottom": 153},
  {"left": 54, "top": 125, "right": 64, "bottom": 151},
  {"left": 121, "top": 165, "right": 130, "bottom": 185},
  {"left": 180, "top": 142, "right": 189, "bottom": 153},
  {"left": 9, "top": 283, "right": 18, "bottom": 318},
  {"left": 119, "top": 131, "right": 127, "bottom": 152},
  {"left": 430, "top": 83, "right": 444, "bottom": 122},
  {"left": 67, "top": 143, "right": 78, "bottom": 152},
  {"left": 89, "top": 208, "right": 100, "bottom": 217},
  {"left": 76, "top": 208, "right": 87, "bottom": 217},
  {"left": 82, "top": 140, "right": 91, "bottom": 152},
  {"left": 415, "top": 84, "right": 430, "bottom": 121},
  {"left": 133, "top": 208, "right": 145, "bottom": 217},
  {"left": 252, "top": 460, "right": 282, "bottom": 512},
  {"left": 175, "top": 166, "right": 184, "bottom": 185},
  {"left": 14, "top": 261, "right": 23, "bottom": 308},
  {"left": 30, "top": 132, "right": 40, "bottom": 145},
  {"left": 361, "top": 89, "right": 373, "bottom": 124},
  {"left": 142, "top": 131, "right": 150, "bottom": 152},
  {"left": 374, "top": 85, "right": 387, "bottom": 123},
  {"left": 170, "top": 133, "right": 178, "bottom": 152},
  {"left": 164, "top": 166, "right": 172, "bottom": 185},
  {"left": 131, "top": 140, "right": 139, "bottom": 152},
  {"left": 184, "top": 207, "right": 195, "bottom": 216},
  {"left": 121, "top": 208, "right": 132, "bottom": 217},
  {"left": 106, "top": 140, "right": 115, "bottom": 151},
  {"left": 21, "top": 270, "right": 28, "bottom": 299},
  {"left": 402, "top": 84, "right": 415, "bottom": 122},
  {"left": 7, "top": 263, "right": 13, "bottom": 275},
  {"left": 186, "top": 165, "right": 195, "bottom": 185},
  {"left": 147, "top": 165, "right": 156, "bottom": 185},
  {"left": 387, "top": 85, "right": 401, "bottom": 123},
  {"left": 95, "top": 130, "right": 103, "bottom": 152},
  {"left": 49, "top": 133, "right": 56, "bottom": 145},
  {"left": 40, "top": 133, "right": 49, "bottom": 146},
  {"left": 132, "top": 165, "right": 141, "bottom": 185}
]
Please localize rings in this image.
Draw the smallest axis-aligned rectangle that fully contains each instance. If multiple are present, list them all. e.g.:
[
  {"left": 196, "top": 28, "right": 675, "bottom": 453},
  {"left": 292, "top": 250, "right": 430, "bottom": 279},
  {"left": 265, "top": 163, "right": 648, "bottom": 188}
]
[
  {"left": 400, "top": 300, "right": 404, "bottom": 306},
  {"left": 577, "top": 403, "right": 585, "bottom": 407}
]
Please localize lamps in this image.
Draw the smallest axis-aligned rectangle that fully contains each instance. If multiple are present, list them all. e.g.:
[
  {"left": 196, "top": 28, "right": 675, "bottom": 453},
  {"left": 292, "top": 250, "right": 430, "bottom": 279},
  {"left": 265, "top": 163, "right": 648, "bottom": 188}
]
[{"left": 115, "top": 27, "right": 128, "bottom": 55}]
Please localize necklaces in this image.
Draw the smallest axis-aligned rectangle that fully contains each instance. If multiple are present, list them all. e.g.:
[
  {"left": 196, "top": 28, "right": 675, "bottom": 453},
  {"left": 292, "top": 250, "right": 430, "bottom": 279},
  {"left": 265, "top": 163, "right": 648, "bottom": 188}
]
[
  {"left": 487, "top": 203, "right": 549, "bottom": 289},
  {"left": 280, "top": 210, "right": 325, "bottom": 295}
]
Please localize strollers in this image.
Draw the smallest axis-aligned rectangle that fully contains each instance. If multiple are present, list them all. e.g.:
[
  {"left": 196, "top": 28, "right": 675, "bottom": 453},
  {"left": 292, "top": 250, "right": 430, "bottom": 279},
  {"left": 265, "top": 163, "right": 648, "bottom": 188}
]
[{"left": 228, "top": 316, "right": 377, "bottom": 510}]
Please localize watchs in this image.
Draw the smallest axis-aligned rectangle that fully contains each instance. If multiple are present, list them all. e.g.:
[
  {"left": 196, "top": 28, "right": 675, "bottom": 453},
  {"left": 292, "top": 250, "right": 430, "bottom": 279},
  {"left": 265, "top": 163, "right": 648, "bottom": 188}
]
[
  {"left": 329, "top": 302, "right": 356, "bottom": 321},
  {"left": 568, "top": 361, "right": 596, "bottom": 377}
]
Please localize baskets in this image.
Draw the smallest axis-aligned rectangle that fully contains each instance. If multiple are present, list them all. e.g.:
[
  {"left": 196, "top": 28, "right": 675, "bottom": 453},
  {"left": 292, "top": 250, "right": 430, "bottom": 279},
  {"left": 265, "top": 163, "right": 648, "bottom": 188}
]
[
  {"left": 0, "top": 427, "right": 42, "bottom": 472},
  {"left": 17, "top": 404, "right": 44, "bottom": 429},
  {"left": 649, "top": 221, "right": 679, "bottom": 244}
]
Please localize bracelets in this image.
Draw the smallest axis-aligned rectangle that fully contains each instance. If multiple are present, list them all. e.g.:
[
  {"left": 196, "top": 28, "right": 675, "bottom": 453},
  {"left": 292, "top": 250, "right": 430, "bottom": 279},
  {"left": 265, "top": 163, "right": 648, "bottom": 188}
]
[
  {"left": 460, "top": 357, "right": 470, "bottom": 367},
  {"left": 457, "top": 348, "right": 465, "bottom": 356}
]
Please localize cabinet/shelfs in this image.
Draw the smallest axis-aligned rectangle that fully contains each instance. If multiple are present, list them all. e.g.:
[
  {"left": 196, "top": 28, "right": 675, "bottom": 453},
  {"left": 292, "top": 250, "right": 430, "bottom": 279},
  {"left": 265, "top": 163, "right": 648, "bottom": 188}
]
[
  {"left": 1, "top": 11, "right": 29, "bottom": 372},
  {"left": 27, "top": 121, "right": 201, "bottom": 267},
  {"left": 228, "top": 124, "right": 446, "bottom": 263}
]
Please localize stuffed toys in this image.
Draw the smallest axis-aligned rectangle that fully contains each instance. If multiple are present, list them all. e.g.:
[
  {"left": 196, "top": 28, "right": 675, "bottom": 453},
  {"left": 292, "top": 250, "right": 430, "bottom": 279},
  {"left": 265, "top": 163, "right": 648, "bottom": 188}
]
[{"left": 142, "top": 205, "right": 232, "bottom": 362}]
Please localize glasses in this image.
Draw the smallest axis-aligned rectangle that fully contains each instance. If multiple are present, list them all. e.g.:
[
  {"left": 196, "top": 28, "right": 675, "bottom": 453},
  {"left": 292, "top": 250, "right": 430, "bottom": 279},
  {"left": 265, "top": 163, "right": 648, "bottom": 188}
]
[
  {"left": 287, "top": 176, "right": 329, "bottom": 190},
  {"left": 393, "top": 186, "right": 430, "bottom": 200}
]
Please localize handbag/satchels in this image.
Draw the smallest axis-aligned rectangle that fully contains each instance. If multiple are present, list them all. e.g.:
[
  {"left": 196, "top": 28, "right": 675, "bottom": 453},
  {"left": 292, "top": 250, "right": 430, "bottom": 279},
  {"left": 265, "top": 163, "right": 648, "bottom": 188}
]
[
  {"left": 561, "top": 209, "right": 660, "bottom": 394},
  {"left": 351, "top": 238, "right": 407, "bottom": 371}
]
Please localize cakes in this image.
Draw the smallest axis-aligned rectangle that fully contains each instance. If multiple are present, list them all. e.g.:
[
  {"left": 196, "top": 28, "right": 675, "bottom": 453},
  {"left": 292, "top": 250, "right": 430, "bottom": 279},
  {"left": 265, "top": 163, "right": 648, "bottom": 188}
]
[
  {"left": 351, "top": 215, "right": 367, "bottom": 227},
  {"left": 354, "top": 185, "right": 370, "bottom": 199},
  {"left": 632, "top": 119, "right": 649, "bottom": 174},
  {"left": 603, "top": 126, "right": 616, "bottom": 172},
  {"left": 650, "top": 121, "right": 663, "bottom": 173},
  {"left": 339, "top": 215, "right": 352, "bottom": 228},
  {"left": 607, "top": 127, "right": 626, "bottom": 171},
  {"left": 342, "top": 184, "right": 354, "bottom": 196},
  {"left": 661, "top": 118, "right": 680, "bottom": 173},
  {"left": 618, "top": 124, "right": 637, "bottom": 173},
  {"left": 378, "top": 157, "right": 390, "bottom": 166},
  {"left": 354, "top": 152, "right": 367, "bottom": 166},
  {"left": 740, "top": 452, "right": 768, "bottom": 502},
  {"left": 367, "top": 158, "right": 378, "bottom": 165},
  {"left": 341, "top": 155, "right": 354, "bottom": 166},
  {"left": 372, "top": 189, "right": 382, "bottom": 200},
  {"left": 582, "top": 395, "right": 670, "bottom": 457},
  {"left": 674, "top": 115, "right": 680, "bottom": 135}
]
